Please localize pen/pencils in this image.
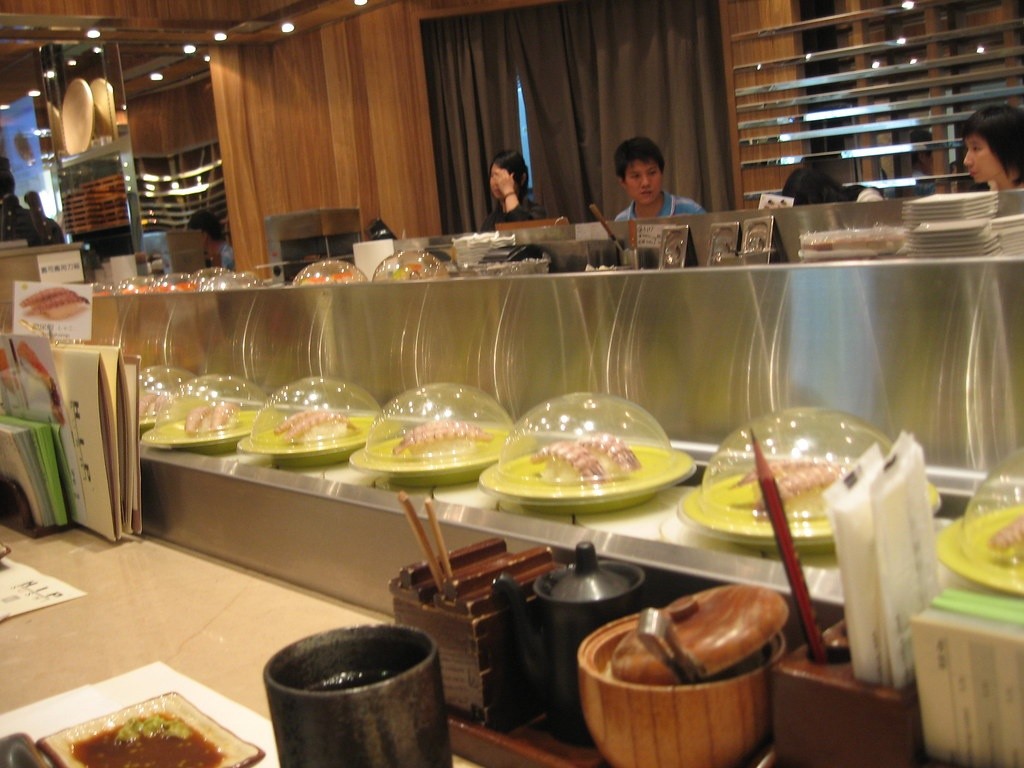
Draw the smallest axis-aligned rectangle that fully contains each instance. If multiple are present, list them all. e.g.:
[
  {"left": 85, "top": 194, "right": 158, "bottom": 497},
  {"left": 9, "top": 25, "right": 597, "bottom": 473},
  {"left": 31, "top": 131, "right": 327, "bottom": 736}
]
[{"left": 747, "top": 425, "right": 830, "bottom": 666}]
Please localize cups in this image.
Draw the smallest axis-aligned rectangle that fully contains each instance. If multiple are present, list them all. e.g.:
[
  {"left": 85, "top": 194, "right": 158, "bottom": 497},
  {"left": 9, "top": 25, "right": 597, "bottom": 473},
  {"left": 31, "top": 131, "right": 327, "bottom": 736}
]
[{"left": 263, "top": 625, "right": 453, "bottom": 768}]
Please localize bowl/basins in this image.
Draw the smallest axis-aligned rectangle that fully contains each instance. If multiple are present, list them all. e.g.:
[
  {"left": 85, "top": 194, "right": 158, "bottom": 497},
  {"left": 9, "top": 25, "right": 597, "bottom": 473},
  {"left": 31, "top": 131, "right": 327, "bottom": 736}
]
[{"left": 576, "top": 585, "right": 789, "bottom": 768}]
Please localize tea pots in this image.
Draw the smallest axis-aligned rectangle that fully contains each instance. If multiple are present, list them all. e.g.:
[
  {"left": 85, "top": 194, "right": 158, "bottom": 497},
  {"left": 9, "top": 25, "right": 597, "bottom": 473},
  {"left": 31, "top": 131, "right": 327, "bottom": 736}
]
[{"left": 496, "top": 541, "right": 649, "bottom": 747}]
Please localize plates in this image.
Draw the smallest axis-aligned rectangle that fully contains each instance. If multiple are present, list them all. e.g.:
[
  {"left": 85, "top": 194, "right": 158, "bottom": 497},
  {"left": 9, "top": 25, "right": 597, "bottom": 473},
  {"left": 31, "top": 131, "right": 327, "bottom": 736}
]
[
  {"left": 479, "top": 445, "right": 697, "bottom": 514},
  {"left": 679, "top": 475, "right": 941, "bottom": 554},
  {"left": 138, "top": 418, "right": 157, "bottom": 428},
  {"left": 37, "top": 691, "right": 265, "bottom": 768},
  {"left": 938, "top": 505, "right": 1024, "bottom": 595},
  {"left": 238, "top": 416, "right": 400, "bottom": 463},
  {"left": 141, "top": 407, "right": 287, "bottom": 456},
  {"left": 903, "top": 191, "right": 1024, "bottom": 257},
  {"left": 349, "top": 429, "right": 536, "bottom": 484}
]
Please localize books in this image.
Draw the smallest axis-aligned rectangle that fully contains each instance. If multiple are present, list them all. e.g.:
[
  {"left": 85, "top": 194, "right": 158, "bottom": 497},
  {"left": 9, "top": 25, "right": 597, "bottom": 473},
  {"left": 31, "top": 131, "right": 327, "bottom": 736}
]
[{"left": 0, "top": 332, "right": 143, "bottom": 541}]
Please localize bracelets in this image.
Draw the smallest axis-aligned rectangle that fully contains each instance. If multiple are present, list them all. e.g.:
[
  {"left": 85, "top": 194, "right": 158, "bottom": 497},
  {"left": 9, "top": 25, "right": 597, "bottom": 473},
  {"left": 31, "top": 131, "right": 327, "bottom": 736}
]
[{"left": 503, "top": 191, "right": 515, "bottom": 201}]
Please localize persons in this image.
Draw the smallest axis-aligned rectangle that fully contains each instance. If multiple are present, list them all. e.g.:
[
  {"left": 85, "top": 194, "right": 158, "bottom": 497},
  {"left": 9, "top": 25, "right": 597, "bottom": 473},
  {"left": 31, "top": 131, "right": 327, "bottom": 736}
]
[
  {"left": 0, "top": 169, "right": 65, "bottom": 248},
  {"left": 881, "top": 130, "right": 937, "bottom": 198},
  {"left": 185, "top": 209, "right": 237, "bottom": 272},
  {"left": 478, "top": 149, "right": 542, "bottom": 263},
  {"left": 962, "top": 104, "right": 1024, "bottom": 191},
  {"left": 783, "top": 167, "right": 883, "bottom": 206},
  {"left": 614, "top": 136, "right": 707, "bottom": 222}
]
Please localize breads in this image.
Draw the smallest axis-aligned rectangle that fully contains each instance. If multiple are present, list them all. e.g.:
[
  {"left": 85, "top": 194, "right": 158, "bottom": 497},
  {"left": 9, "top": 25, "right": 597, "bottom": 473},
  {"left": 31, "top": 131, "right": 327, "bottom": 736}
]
[{"left": 21, "top": 289, "right": 91, "bottom": 319}]
